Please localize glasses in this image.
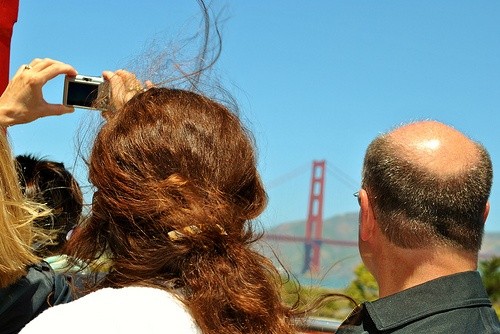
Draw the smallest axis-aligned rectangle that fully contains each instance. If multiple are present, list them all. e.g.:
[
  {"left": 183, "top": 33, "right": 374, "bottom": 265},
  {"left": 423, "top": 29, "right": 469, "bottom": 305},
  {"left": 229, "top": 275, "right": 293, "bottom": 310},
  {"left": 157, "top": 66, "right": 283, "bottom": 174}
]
[{"left": 353, "top": 190, "right": 362, "bottom": 200}]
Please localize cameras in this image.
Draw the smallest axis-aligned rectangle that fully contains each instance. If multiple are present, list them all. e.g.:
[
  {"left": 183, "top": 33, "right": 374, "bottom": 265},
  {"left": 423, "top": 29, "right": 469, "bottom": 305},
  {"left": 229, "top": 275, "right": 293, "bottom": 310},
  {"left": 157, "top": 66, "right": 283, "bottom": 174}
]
[{"left": 63, "top": 73, "right": 112, "bottom": 111}]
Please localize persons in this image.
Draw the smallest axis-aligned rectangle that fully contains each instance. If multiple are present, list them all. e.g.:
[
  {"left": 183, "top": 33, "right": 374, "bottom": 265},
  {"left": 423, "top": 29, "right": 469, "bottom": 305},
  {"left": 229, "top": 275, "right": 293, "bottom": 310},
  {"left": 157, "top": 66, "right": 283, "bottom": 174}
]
[
  {"left": 14, "top": 153, "right": 91, "bottom": 275},
  {"left": 16, "top": 0, "right": 361, "bottom": 334},
  {"left": 0, "top": 58, "right": 153, "bottom": 334},
  {"left": 333, "top": 119, "right": 500, "bottom": 334}
]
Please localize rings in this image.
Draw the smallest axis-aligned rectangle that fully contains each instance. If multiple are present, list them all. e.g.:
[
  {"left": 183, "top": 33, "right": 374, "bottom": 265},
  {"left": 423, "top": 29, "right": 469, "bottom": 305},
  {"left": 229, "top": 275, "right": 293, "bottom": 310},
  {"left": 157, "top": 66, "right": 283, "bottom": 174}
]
[{"left": 25, "top": 66, "right": 33, "bottom": 69}]
